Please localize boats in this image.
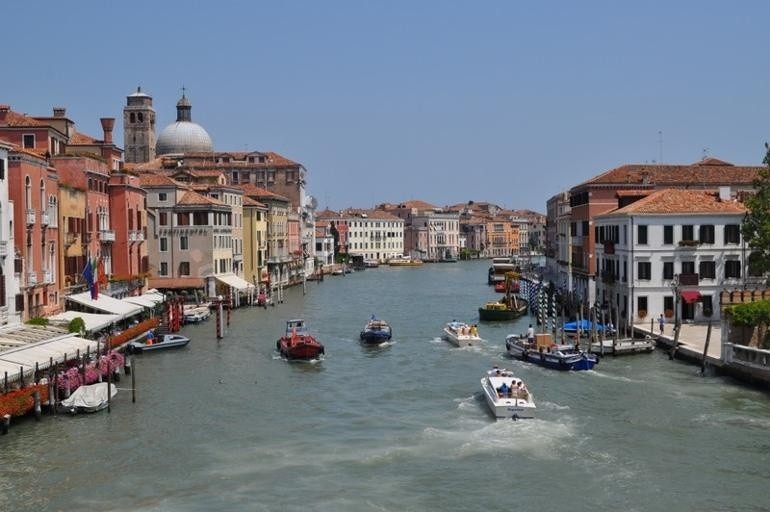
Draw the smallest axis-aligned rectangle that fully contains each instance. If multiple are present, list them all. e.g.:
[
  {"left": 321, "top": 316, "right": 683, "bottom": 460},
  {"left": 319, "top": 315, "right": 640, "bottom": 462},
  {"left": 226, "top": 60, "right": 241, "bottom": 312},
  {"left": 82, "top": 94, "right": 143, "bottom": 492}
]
[
  {"left": 276, "top": 318, "right": 325, "bottom": 360},
  {"left": 387, "top": 255, "right": 423, "bottom": 267},
  {"left": 505, "top": 333, "right": 599, "bottom": 373},
  {"left": 443, "top": 321, "right": 481, "bottom": 347},
  {"left": 589, "top": 336, "right": 656, "bottom": 356},
  {"left": 487, "top": 257, "right": 523, "bottom": 286},
  {"left": 495, "top": 279, "right": 520, "bottom": 293},
  {"left": 478, "top": 271, "right": 530, "bottom": 322},
  {"left": 364, "top": 258, "right": 379, "bottom": 268},
  {"left": 59, "top": 381, "right": 118, "bottom": 415},
  {"left": 360, "top": 314, "right": 392, "bottom": 344},
  {"left": 127, "top": 333, "right": 191, "bottom": 355},
  {"left": 184, "top": 306, "right": 212, "bottom": 323},
  {"left": 333, "top": 266, "right": 351, "bottom": 275},
  {"left": 480, "top": 368, "right": 537, "bottom": 420},
  {"left": 559, "top": 318, "right": 615, "bottom": 338},
  {"left": 250, "top": 297, "right": 270, "bottom": 307}
]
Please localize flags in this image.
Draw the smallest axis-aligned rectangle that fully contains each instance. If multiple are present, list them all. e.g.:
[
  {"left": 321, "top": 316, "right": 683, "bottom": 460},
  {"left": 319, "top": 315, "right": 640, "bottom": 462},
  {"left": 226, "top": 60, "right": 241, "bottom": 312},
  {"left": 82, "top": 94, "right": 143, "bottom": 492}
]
[
  {"left": 90, "top": 259, "right": 99, "bottom": 299},
  {"left": 82, "top": 258, "right": 96, "bottom": 295}
]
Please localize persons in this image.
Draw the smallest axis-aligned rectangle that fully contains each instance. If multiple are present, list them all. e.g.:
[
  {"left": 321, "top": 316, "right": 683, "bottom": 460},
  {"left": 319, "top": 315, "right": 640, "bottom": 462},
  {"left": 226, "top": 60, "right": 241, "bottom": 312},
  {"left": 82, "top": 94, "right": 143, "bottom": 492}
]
[
  {"left": 527, "top": 323, "right": 534, "bottom": 343},
  {"left": 549, "top": 280, "right": 555, "bottom": 292},
  {"left": 573, "top": 335, "right": 579, "bottom": 351},
  {"left": 657, "top": 314, "right": 665, "bottom": 335},
  {"left": 450, "top": 319, "right": 478, "bottom": 337},
  {"left": 145, "top": 330, "right": 154, "bottom": 345},
  {"left": 499, "top": 380, "right": 523, "bottom": 398}
]
